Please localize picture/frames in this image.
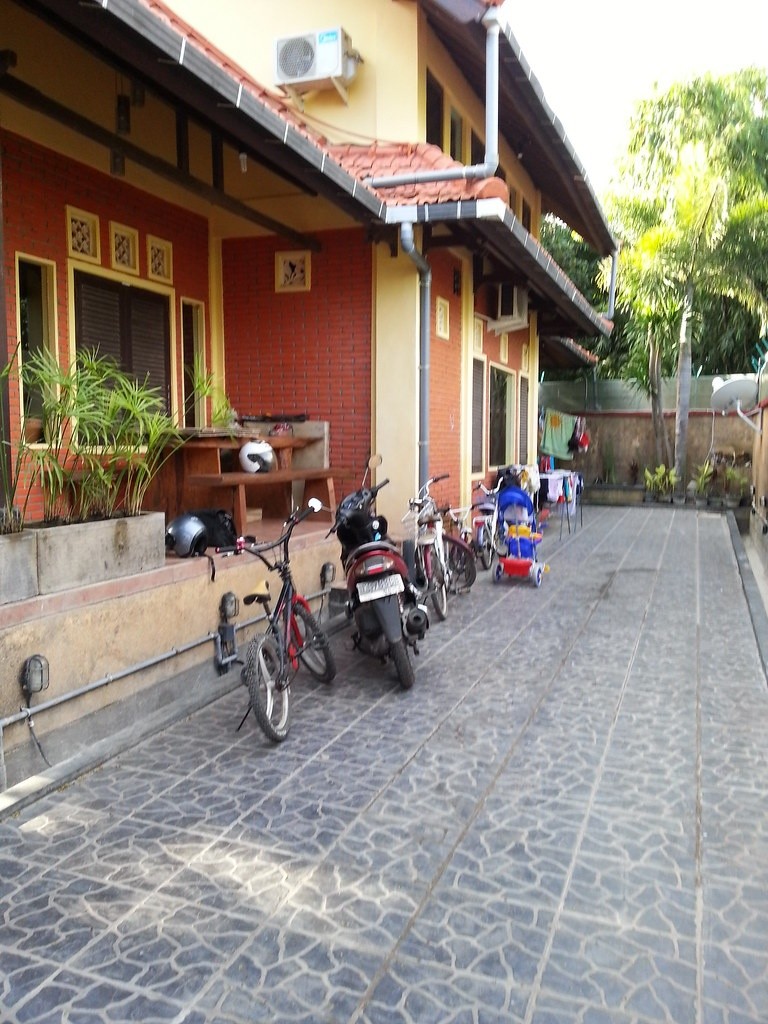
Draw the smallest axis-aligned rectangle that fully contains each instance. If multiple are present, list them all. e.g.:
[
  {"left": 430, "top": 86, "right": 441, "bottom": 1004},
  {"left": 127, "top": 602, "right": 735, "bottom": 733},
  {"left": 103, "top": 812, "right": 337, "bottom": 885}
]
[
  {"left": 435, "top": 296, "right": 450, "bottom": 341},
  {"left": 275, "top": 250, "right": 311, "bottom": 292}
]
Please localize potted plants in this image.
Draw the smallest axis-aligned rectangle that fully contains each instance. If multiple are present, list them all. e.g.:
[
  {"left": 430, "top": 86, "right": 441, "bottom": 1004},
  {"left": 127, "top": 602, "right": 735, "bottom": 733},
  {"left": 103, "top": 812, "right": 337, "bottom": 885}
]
[
  {"left": 644, "top": 460, "right": 752, "bottom": 508},
  {"left": 0, "top": 341, "right": 238, "bottom": 606}
]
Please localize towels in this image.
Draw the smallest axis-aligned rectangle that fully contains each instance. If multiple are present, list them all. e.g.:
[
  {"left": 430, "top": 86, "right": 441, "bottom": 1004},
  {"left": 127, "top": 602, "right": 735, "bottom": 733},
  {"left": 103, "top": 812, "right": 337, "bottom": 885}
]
[{"left": 539, "top": 407, "right": 578, "bottom": 460}]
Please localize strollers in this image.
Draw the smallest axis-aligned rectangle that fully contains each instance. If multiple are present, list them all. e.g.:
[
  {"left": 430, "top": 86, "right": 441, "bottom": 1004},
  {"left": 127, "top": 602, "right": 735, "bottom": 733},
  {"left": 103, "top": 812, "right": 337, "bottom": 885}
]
[{"left": 492, "top": 484, "right": 550, "bottom": 588}]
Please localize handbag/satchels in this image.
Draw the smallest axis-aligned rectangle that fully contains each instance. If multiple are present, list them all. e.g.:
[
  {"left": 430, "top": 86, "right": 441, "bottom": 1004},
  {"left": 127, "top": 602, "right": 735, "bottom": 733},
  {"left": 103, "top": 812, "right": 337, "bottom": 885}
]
[{"left": 189, "top": 508, "right": 240, "bottom": 550}]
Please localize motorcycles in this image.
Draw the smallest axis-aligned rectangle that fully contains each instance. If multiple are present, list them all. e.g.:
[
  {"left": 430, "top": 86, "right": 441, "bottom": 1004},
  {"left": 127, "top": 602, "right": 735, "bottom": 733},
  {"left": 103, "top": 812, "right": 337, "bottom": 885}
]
[{"left": 307, "top": 453, "right": 430, "bottom": 690}]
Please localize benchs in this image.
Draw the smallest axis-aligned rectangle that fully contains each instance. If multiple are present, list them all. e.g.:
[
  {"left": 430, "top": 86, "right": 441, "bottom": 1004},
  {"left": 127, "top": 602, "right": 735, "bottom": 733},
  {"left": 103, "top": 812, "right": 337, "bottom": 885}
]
[{"left": 191, "top": 467, "right": 355, "bottom": 539}]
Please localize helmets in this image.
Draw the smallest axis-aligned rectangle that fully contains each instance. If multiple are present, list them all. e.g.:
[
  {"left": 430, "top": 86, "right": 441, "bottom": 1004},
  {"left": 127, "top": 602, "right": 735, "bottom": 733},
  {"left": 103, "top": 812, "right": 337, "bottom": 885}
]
[
  {"left": 239, "top": 440, "right": 279, "bottom": 474},
  {"left": 165, "top": 514, "right": 210, "bottom": 558}
]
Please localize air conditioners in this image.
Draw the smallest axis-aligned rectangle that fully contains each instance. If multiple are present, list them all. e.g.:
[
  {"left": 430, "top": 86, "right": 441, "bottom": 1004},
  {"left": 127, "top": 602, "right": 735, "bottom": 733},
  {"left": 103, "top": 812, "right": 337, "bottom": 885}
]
[{"left": 270, "top": 26, "right": 357, "bottom": 92}]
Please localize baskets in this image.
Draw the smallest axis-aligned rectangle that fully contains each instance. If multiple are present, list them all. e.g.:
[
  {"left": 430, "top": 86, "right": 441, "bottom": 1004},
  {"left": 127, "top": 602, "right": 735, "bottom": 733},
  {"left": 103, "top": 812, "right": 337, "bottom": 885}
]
[{"left": 402, "top": 498, "right": 434, "bottom": 541}]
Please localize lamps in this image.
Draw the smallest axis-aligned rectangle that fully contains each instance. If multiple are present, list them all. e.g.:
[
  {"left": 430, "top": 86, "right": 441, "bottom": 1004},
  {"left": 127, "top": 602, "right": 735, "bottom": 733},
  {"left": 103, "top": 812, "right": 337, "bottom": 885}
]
[
  {"left": 239, "top": 151, "right": 248, "bottom": 174},
  {"left": 218, "top": 593, "right": 239, "bottom": 618},
  {"left": 23, "top": 654, "right": 50, "bottom": 692},
  {"left": 320, "top": 563, "right": 336, "bottom": 582}
]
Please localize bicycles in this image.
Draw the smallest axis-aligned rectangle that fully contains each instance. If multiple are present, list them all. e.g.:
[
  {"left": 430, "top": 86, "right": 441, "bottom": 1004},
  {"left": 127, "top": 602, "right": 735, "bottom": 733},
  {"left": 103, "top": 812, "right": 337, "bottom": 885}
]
[
  {"left": 402, "top": 471, "right": 476, "bottom": 621},
  {"left": 434, "top": 500, "right": 485, "bottom": 588},
  {"left": 469, "top": 473, "right": 512, "bottom": 570},
  {"left": 215, "top": 504, "right": 338, "bottom": 745}
]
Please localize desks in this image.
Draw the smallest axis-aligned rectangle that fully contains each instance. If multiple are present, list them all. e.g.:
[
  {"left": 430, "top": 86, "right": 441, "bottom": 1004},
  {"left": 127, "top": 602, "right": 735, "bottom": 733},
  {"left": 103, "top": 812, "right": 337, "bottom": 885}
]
[{"left": 146, "top": 438, "right": 308, "bottom": 525}]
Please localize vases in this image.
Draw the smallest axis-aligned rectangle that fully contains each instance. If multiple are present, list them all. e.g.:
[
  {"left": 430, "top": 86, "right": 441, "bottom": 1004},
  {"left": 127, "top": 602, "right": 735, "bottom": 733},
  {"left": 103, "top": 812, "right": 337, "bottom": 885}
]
[{"left": 25, "top": 419, "right": 43, "bottom": 444}]
